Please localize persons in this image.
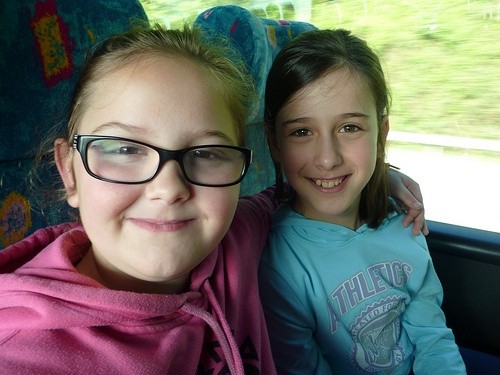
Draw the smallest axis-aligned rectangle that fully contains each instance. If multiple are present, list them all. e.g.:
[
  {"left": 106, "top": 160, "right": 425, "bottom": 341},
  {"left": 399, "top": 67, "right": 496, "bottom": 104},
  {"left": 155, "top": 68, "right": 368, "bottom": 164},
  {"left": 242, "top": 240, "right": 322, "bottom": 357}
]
[
  {"left": 0, "top": 21, "right": 429, "bottom": 375},
  {"left": 256, "top": 28, "right": 500, "bottom": 375}
]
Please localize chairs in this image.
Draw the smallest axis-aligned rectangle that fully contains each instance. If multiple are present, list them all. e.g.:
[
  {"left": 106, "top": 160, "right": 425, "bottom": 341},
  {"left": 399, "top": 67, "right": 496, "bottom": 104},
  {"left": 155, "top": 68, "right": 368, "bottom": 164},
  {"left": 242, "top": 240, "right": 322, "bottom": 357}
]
[{"left": 0, "top": 0, "right": 320, "bottom": 249}]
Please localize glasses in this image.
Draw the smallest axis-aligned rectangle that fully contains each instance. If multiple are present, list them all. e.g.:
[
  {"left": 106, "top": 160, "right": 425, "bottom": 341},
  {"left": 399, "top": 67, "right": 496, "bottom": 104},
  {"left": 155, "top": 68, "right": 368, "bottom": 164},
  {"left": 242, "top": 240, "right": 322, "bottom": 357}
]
[{"left": 71, "top": 134, "right": 255, "bottom": 187}]
